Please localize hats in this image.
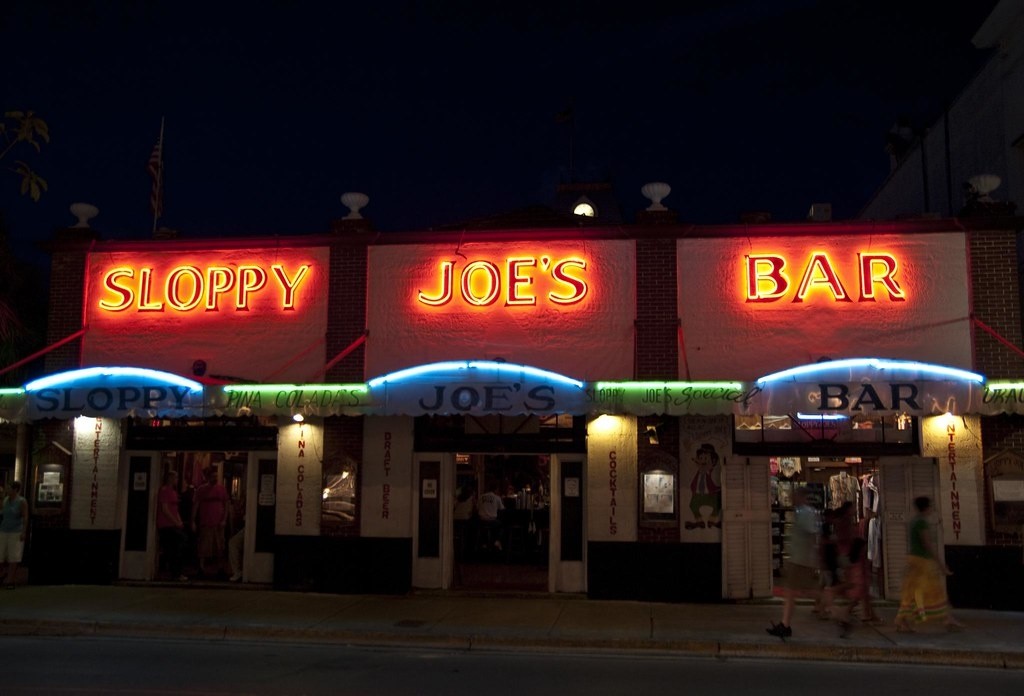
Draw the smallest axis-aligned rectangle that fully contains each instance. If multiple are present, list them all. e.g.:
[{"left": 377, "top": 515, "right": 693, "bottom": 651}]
[{"left": 203, "top": 465, "right": 218, "bottom": 475}]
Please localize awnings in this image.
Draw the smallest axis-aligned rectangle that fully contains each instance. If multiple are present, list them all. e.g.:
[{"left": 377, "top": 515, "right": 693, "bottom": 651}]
[{"left": 0, "top": 357, "right": 1024, "bottom": 424}]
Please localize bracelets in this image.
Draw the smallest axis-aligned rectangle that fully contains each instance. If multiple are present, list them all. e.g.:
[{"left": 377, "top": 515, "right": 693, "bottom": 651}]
[{"left": 191, "top": 519, "right": 195, "bottom": 521}]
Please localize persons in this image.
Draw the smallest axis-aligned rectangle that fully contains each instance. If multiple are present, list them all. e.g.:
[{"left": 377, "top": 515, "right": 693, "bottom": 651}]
[
  {"left": 191, "top": 465, "right": 230, "bottom": 578},
  {"left": 764, "top": 487, "right": 885, "bottom": 640},
  {"left": 894, "top": 496, "right": 966, "bottom": 634},
  {"left": 0, "top": 481, "right": 28, "bottom": 586},
  {"left": 230, "top": 515, "right": 245, "bottom": 581},
  {"left": 476, "top": 483, "right": 504, "bottom": 551},
  {"left": 158, "top": 470, "right": 188, "bottom": 582},
  {"left": 454, "top": 486, "right": 476, "bottom": 539}
]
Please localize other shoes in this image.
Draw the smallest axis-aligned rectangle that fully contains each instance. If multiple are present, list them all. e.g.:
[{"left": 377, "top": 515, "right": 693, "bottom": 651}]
[
  {"left": 6, "top": 581, "right": 16, "bottom": 590},
  {"left": 229, "top": 569, "right": 241, "bottom": 580},
  {"left": 494, "top": 540, "right": 503, "bottom": 552},
  {"left": 480, "top": 543, "right": 487, "bottom": 549},
  {"left": 897, "top": 626, "right": 919, "bottom": 635},
  {"left": 766, "top": 622, "right": 792, "bottom": 637},
  {"left": 945, "top": 616, "right": 965, "bottom": 628},
  {"left": 839, "top": 625, "right": 857, "bottom": 639}
]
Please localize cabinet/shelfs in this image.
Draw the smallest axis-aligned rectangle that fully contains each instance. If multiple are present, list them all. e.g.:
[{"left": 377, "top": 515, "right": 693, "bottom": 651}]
[{"left": 771, "top": 507, "right": 799, "bottom": 579}]
